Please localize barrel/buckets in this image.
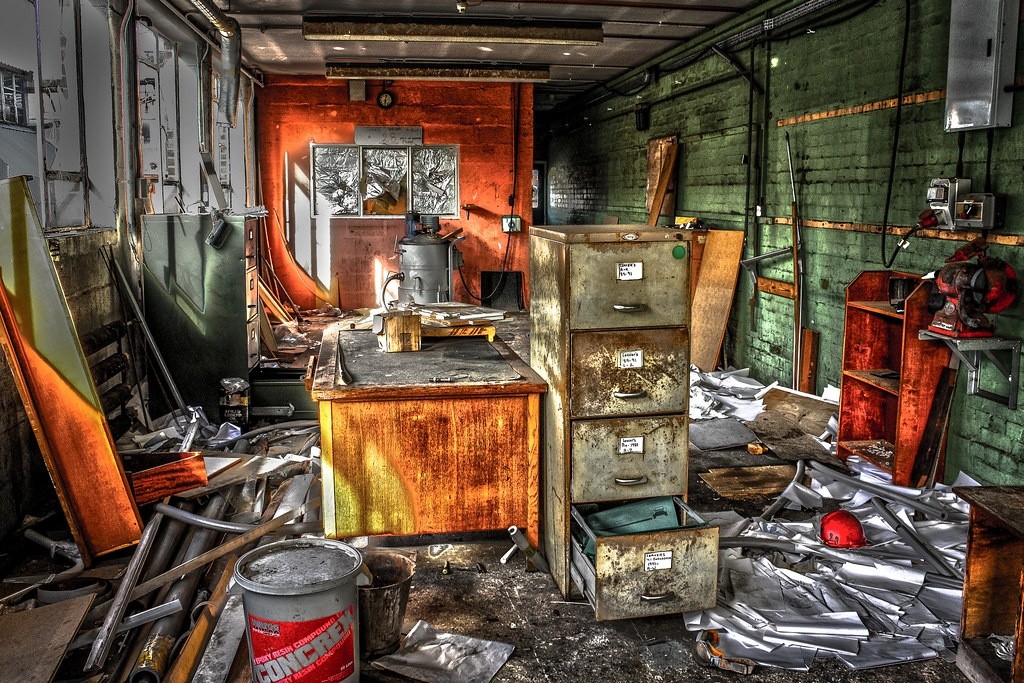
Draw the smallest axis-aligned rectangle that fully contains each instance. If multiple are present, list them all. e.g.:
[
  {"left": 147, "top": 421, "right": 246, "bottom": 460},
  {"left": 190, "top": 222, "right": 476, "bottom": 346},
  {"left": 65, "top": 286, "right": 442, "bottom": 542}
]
[
  {"left": 234, "top": 539, "right": 363, "bottom": 683},
  {"left": 358, "top": 547, "right": 415, "bottom": 660}
]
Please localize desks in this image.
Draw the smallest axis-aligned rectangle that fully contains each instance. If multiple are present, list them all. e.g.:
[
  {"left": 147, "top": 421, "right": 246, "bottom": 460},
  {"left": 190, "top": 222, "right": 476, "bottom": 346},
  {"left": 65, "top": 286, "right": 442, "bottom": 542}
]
[{"left": 951, "top": 485, "right": 1024, "bottom": 683}]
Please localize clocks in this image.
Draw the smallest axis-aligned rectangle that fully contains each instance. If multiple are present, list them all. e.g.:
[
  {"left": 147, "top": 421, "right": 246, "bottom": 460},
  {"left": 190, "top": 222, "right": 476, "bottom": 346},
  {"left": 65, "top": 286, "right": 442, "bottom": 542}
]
[{"left": 377, "top": 91, "right": 395, "bottom": 108}]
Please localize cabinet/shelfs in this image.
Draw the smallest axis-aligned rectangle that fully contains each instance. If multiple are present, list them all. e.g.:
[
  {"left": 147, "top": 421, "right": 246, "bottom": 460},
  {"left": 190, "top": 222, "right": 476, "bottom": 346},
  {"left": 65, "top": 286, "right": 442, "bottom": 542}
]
[
  {"left": 139, "top": 211, "right": 261, "bottom": 424},
  {"left": 311, "top": 323, "right": 547, "bottom": 552},
  {"left": 835, "top": 270, "right": 952, "bottom": 488},
  {"left": 42, "top": 226, "right": 132, "bottom": 445},
  {"left": 529, "top": 226, "right": 720, "bottom": 622}
]
